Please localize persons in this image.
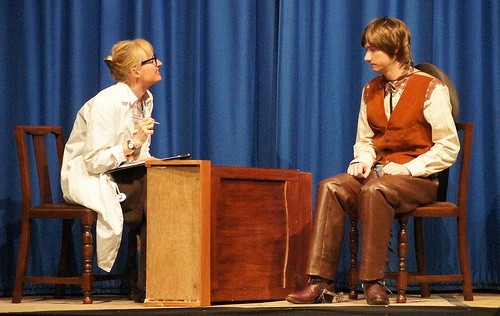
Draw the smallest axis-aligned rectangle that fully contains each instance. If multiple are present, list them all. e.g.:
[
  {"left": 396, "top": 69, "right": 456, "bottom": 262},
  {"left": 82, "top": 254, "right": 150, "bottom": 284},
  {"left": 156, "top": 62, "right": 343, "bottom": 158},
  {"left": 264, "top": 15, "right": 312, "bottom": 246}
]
[
  {"left": 61, "top": 37, "right": 163, "bottom": 297},
  {"left": 287, "top": 16, "right": 460, "bottom": 305}
]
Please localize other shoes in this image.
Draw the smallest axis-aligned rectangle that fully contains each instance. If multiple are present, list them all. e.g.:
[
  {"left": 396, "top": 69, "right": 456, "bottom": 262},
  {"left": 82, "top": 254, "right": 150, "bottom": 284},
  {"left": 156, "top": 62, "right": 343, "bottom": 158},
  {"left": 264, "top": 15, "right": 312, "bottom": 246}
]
[{"left": 128, "top": 286, "right": 146, "bottom": 303}]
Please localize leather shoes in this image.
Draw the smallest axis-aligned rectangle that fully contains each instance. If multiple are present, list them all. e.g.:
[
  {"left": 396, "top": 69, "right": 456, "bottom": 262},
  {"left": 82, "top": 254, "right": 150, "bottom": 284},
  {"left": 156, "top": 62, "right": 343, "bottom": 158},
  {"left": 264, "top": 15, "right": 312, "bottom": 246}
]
[
  {"left": 286, "top": 278, "right": 335, "bottom": 303},
  {"left": 364, "top": 281, "right": 393, "bottom": 305}
]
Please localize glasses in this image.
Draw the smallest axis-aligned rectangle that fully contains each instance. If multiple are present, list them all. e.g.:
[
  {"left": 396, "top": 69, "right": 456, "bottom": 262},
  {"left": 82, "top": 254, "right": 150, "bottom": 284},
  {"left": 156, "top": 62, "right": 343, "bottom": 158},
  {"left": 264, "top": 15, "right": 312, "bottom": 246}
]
[{"left": 141, "top": 53, "right": 157, "bottom": 67}]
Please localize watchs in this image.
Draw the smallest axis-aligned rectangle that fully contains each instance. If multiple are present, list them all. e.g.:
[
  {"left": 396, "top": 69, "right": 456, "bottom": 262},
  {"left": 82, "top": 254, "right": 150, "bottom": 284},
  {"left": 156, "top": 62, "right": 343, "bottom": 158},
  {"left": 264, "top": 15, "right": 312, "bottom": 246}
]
[{"left": 127, "top": 139, "right": 137, "bottom": 151}]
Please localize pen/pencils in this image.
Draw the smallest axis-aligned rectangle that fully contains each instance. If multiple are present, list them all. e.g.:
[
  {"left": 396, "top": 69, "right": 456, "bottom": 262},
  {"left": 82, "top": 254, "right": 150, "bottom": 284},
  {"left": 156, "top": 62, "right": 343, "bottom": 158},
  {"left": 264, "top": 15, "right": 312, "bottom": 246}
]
[{"left": 132, "top": 115, "right": 160, "bottom": 125}]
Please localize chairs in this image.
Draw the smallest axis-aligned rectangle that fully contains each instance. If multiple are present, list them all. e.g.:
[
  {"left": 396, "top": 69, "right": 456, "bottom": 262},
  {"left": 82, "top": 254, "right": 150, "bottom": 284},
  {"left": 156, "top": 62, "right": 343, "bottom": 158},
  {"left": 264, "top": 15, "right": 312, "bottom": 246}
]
[
  {"left": 12, "top": 127, "right": 139, "bottom": 305},
  {"left": 350, "top": 122, "right": 474, "bottom": 304}
]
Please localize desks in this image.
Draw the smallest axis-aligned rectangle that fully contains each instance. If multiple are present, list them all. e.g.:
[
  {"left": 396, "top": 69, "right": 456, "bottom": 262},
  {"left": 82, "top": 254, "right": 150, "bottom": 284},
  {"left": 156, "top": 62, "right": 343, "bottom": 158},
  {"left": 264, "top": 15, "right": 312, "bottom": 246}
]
[{"left": 143, "top": 159, "right": 314, "bottom": 307}]
